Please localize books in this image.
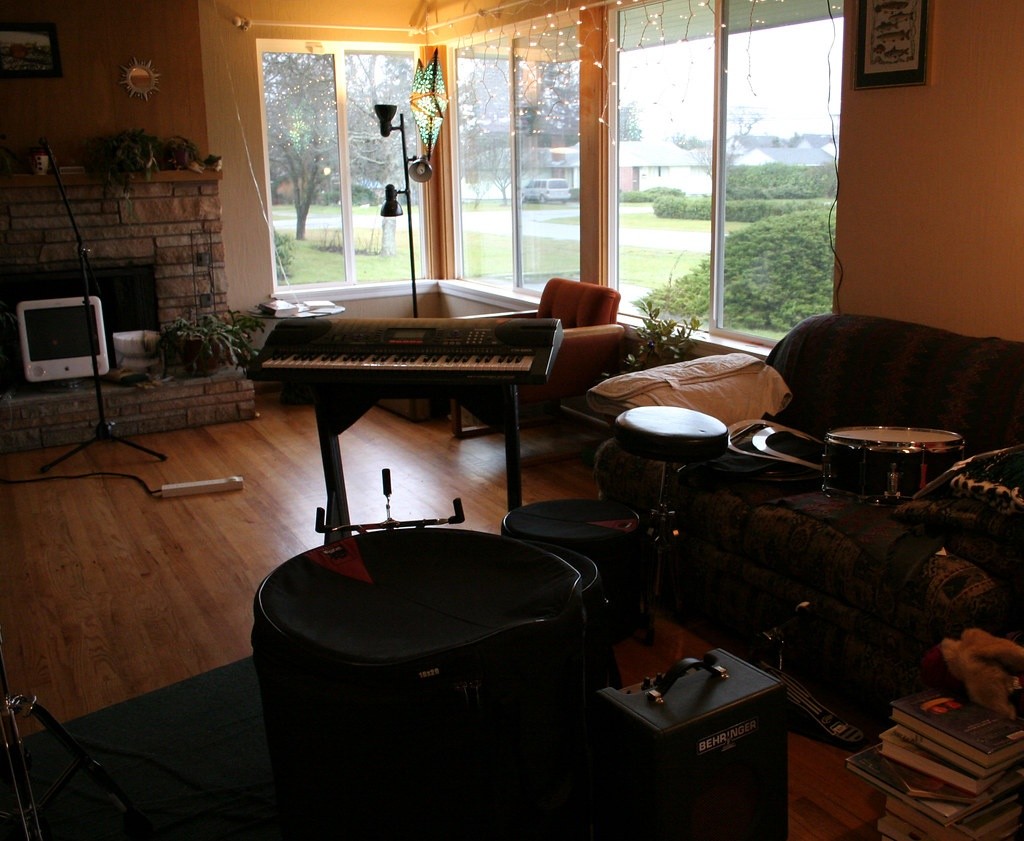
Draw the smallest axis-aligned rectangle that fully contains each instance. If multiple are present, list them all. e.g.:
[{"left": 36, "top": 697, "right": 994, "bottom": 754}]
[
  {"left": 846, "top": 688, "right": 1024, "bottom": 841},
  {"left": 258, "top": 295, "right": 344, "bottom": 317}
]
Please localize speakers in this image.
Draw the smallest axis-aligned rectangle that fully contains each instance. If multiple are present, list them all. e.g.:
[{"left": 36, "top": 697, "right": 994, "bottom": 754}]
[{"left": 596, "top": 648, "right": 788, "bottom": 840}]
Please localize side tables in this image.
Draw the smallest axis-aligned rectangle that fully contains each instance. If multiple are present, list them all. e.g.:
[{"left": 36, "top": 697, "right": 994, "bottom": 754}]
[{"left": 543, "top": 396, "right": 614, "bottom": 439}]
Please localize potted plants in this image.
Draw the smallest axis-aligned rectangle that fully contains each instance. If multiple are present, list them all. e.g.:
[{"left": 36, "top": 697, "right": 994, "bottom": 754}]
[
  {"left": 159, "top": 134, "right": 206, "bottom": 170},
  {"left": 158, "top": 310, "right": 265, "bottom": 378},
  {"left": 83, "top": 127, "right": 163, "bottom": 225}
]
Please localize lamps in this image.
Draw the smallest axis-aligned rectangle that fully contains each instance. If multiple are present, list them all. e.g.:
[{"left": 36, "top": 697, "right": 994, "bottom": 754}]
[{"left": 374, "top": 103, "right": 433, "bottom": 318}]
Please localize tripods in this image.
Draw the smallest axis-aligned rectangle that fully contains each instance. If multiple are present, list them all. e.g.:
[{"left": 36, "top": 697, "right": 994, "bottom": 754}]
[
  {"left": 0, "top": 650, "right": 154, "bottom": 841},
  {"left": 40, "top": 139, "right": 167, "bottom": 473}
]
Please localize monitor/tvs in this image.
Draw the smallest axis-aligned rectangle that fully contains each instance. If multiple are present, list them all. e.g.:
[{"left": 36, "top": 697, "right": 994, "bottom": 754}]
[{"left": 17, "top": 296, "right": 109, "bottom": 382}]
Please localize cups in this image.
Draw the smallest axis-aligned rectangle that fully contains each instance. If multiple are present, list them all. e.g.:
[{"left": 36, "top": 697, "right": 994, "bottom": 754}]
[{"left": 32, "top": 156, "right": 49, "bottom": 175}]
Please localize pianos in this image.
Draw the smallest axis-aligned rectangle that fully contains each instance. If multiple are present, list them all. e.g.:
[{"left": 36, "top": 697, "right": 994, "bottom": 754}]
[{"left": 246, "top": 318, "right": 565, "bottom": 545}]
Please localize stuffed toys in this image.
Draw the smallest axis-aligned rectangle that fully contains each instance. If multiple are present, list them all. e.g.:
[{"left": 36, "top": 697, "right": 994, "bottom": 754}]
[{"left": 923, "top": 626, "right": 1024, "bottom": 718}]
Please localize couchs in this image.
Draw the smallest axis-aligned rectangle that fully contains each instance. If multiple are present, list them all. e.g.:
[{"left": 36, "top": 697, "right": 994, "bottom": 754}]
[{"left": 592, "top": 313, "right": 1024, "bottom": 731}]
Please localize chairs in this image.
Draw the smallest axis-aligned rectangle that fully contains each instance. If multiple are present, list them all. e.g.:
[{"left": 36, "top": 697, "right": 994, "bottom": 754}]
[{"left": 451, "top": 278, "right": 626, "bottom": 468}]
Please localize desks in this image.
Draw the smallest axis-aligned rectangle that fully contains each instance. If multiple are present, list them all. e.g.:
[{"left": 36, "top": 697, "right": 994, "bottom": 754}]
[{"left": 247, "top": 304, "right": 345, "bottom": 319}]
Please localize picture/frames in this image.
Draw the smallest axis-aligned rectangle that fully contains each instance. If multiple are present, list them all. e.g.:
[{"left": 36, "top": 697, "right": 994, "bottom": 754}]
[
  {"left": 0, "top": 21, "right": 64, "bottom": 80},
  {"left": 849, "top": 0, "right": 937, "bottom": 92}
]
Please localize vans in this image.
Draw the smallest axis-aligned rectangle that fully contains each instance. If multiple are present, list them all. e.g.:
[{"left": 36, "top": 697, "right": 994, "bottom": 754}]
[{"left": 523, "top": 178, "right": 571, "bottom": 205}]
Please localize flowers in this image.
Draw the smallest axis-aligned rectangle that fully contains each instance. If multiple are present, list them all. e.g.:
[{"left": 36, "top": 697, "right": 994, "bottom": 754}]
[{"left": 621, "top": 298, "right": 704, "bottom": 371}]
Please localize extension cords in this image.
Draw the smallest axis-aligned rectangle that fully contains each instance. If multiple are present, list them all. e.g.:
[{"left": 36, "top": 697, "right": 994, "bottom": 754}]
[{"left": 161, "top": 476, "right": 244, "bottom": 498}]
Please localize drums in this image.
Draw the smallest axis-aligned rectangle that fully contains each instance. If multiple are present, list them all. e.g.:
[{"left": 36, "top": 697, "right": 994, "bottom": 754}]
[{"left": 823, "top": 425, "right": 966, "bottom": 508}]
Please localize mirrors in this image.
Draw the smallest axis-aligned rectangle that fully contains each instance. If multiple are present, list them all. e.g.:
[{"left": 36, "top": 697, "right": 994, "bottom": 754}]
[{"left": 118, "top": 56, "right": 162, "bottom": 102}]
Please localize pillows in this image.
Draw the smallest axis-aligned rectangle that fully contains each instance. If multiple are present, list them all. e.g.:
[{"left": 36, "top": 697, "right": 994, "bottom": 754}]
[{"left": 890, "top": 495, "right": 1024, "bottom": 546}]
